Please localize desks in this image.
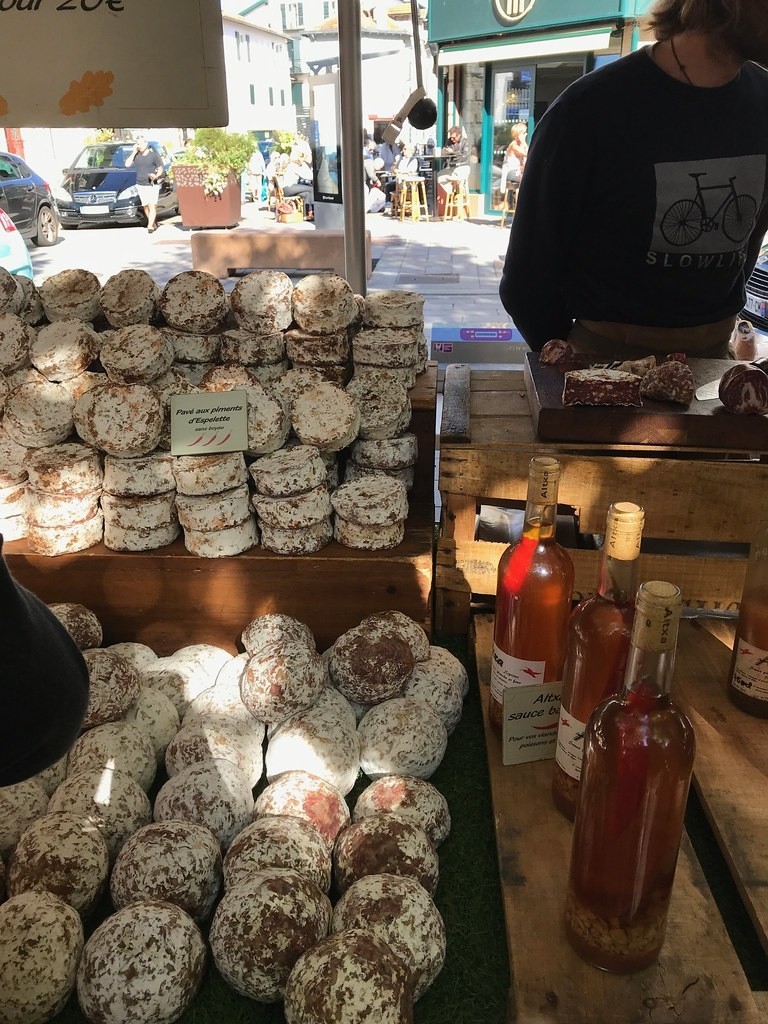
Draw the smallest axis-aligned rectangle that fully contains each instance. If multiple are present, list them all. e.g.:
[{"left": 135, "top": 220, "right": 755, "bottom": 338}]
[{"left": 375, "top": 171, "right": 419, "bottom": 212}]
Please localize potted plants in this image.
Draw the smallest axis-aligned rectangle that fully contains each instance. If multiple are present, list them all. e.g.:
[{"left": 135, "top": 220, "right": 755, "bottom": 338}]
[{"left": 172, "top": 126, "right": 253, "bottom": 231}]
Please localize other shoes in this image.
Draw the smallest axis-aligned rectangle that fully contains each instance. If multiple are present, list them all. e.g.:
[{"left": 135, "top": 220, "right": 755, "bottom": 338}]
[{"left": 148, "top": 224, "right": 158, "bottom": 233}]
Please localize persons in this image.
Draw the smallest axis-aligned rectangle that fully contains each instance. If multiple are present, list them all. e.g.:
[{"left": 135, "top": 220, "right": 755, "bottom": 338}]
[
  {"left": 437, "top": 126, "right": 470, "bottom": 203},
  {"left": 500, "top": 123, "right": 529, "bottom": 183},
  {"left": 500, "top": 0, "right": 768, "bottom": 358},
  {"left": 124, "top": 137, "right": 165, "bottom": 232},
  {"left": 364, "top": 140, "right": 417, "bottom": 216},
  {"left": 160, "top": 146, "right": 171, "bottom": 165},
  {"left": 245, "top": 136, "right": 314, "bottom": 221}
]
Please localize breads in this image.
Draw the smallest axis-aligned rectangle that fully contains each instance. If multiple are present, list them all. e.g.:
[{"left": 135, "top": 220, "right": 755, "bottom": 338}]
[
  {"left": 0, "top": 267, "right": 429, "bottom": 552},
  {"left": 0, "top": 597, "right": 468, "bottom": 1024},
  {"left": 538, "top": 341, "right": 768, "bottom": 416}
]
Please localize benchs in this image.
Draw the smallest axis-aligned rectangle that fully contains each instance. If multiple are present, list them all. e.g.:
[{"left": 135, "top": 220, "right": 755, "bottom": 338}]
[{"left": 190, "top": 229, "right": 372, "bottom": 287}]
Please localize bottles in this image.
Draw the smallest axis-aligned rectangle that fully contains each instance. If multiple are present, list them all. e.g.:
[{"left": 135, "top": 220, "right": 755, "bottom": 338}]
[
  {"left": 488, "top": 455, "right": 576, "bottom": 739},
  {"left": 551, "top": 498, "right": 645, "bottom": 825},
  {"left": 563, "top": 579, "right": 696, "bottom": 973},
  {"left": 725, "top": 521, "right": 768, "bottom": 719}
]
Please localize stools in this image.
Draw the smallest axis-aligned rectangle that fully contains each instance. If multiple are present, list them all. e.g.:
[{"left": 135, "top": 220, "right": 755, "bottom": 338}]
[{"left": 390, "top": 175, "right": 521, "bottom": 228}]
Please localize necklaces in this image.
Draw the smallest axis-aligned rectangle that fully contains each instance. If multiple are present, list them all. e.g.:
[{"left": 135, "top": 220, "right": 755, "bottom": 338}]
[{"left": 671, "top": 37, "right": 692, "bottom": 85}]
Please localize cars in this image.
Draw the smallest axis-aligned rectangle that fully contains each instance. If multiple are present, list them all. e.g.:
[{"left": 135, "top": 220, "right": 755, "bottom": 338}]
[
  {"left": 0, "top": 152, "right": 61, "bottom": 248},
  {"left": 56, "top": 140, "right": 178, "bottom": 229}
]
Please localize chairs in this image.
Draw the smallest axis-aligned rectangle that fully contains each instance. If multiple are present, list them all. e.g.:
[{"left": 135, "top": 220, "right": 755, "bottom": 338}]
[
  {"left": 264, "top": 173, "right": 304, "bottom": 223},
  {"left": 99, "top": 161, "right": 118, "bottom": 167}
]
